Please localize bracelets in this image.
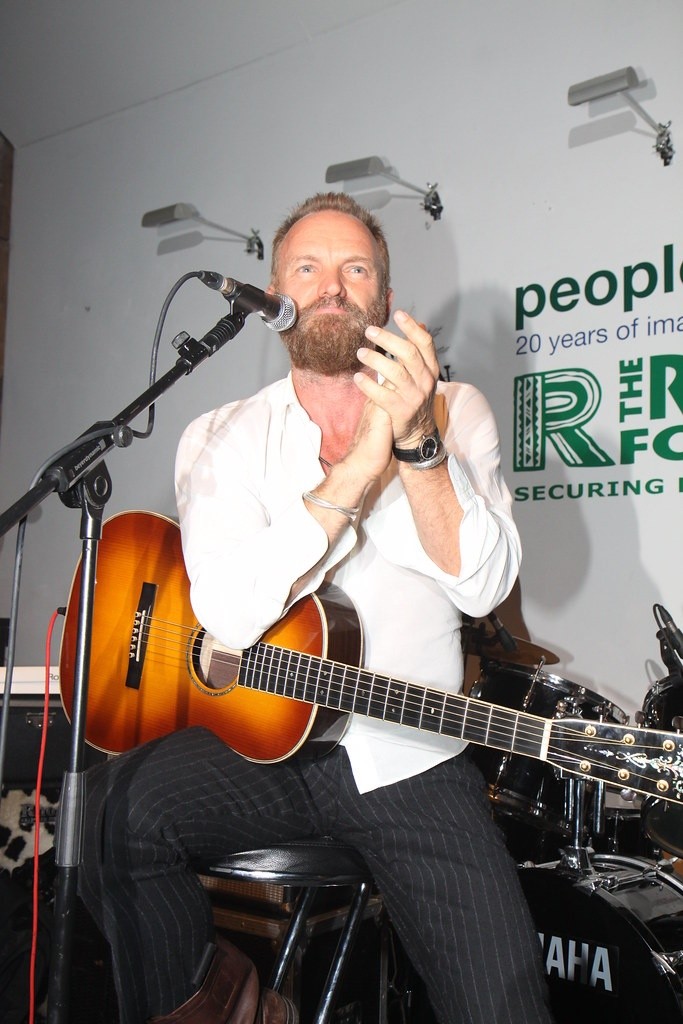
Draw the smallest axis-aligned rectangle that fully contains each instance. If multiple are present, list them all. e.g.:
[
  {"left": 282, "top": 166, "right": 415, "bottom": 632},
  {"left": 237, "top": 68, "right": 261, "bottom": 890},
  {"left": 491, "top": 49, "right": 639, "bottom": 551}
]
[
  {"left": 410, "top": 446, "right": 447, "bottom": 470},
  {"left": 304, "top": 491, "right": 361, "bottom": 522}
]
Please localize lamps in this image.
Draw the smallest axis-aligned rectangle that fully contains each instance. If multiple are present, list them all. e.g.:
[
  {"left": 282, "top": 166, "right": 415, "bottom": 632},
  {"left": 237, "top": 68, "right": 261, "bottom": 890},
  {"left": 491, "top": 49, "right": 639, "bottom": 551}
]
[
  {"left": 324, "top": 157, "right": 444, "bottom": 223},
  {"left": 141, "top": 202, "right": 262, "bottom": 260},
  {"left": 566, "top": 65, "right": 676, "bottom": 167}
]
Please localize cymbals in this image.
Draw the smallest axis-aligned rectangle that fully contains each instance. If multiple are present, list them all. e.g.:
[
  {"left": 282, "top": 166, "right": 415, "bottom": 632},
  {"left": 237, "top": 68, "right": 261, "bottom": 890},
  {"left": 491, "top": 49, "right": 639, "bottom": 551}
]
[{"left": 459, "top": 623, "right": 560, "bottom": 668}]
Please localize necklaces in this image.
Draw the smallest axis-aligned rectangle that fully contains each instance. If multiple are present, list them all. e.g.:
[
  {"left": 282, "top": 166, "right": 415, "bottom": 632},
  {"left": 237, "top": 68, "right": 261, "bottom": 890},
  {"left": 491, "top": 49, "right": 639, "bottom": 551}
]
[{"left": 319, "top": 453, "right": 338, "bottom": 473}]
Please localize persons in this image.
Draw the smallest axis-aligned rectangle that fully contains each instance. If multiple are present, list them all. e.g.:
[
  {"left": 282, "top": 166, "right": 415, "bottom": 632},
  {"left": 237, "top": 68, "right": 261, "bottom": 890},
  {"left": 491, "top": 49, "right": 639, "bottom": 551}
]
[{"left": 67, "top": 193, "right": 551, "bottom": 1023}]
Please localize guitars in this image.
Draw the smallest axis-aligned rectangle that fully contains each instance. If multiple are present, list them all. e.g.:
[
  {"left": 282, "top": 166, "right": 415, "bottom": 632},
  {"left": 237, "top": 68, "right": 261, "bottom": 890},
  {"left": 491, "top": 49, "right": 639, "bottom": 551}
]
[{"left": 57, "top": 510, "right": 682, "bottom": 806}]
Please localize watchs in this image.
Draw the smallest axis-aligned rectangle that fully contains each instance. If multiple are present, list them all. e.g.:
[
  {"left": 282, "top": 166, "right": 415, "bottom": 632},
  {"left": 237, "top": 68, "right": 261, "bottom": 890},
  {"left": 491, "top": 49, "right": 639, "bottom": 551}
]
[{"left": 392, "top": 426, "right": 441, "bottom": 463}]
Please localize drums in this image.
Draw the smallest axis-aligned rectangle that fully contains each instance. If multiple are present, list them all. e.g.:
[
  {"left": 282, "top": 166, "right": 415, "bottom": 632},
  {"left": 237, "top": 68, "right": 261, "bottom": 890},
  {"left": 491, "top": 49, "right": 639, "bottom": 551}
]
[
  {"left": 464, "top": 659, "right": 630, "bottom": 842},
  {"left": 634, "top": 673, "right": 683, "bottom": 860},
  {"left": 406, "top": 845, "right": 683, "bottom": 1023}
]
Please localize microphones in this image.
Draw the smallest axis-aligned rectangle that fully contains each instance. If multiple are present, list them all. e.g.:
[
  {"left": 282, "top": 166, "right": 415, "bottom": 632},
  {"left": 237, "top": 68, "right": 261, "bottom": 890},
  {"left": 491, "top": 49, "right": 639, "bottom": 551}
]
[
  {"left": 197, "top": 271, "right": 299, "bottom": 333},
  {"left": 488, "top": 611, "right": 517, "bottom": 654},
  {"left": 659, "top": 606, "right": 683, "bottom": 661}
]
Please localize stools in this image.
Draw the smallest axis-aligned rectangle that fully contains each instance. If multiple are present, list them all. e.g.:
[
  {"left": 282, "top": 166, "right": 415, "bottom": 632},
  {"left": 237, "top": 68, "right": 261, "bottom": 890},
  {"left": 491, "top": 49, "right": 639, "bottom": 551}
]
[{"left": 199, "top": 841, "right": 397, "bottom": 1024}]
